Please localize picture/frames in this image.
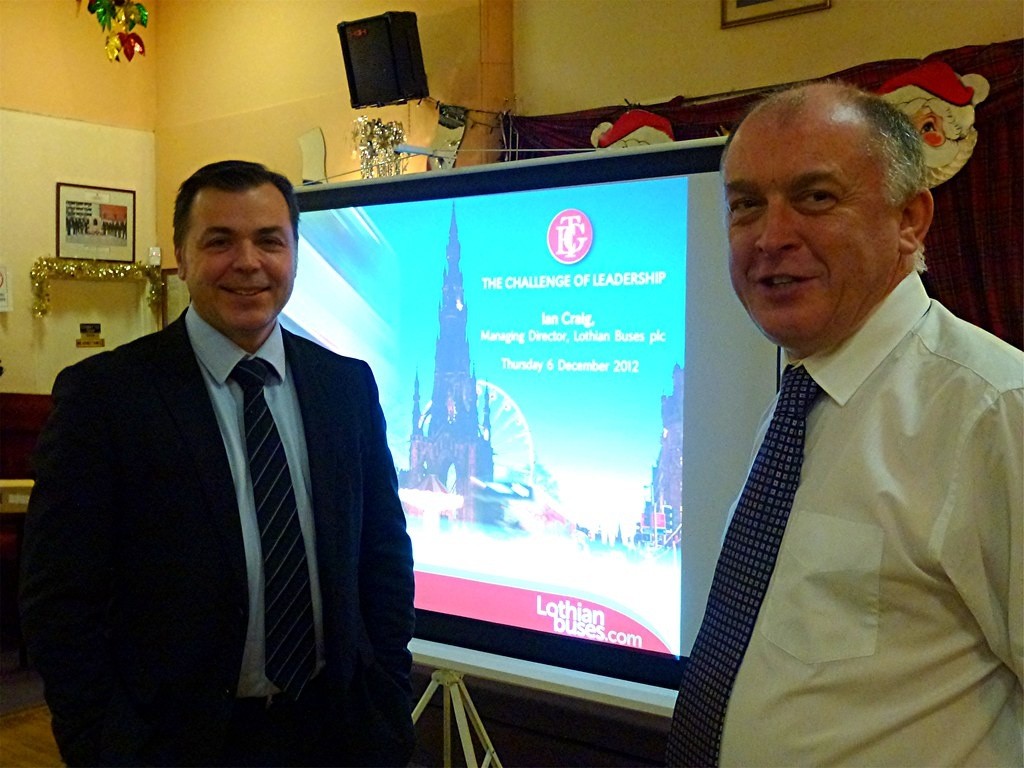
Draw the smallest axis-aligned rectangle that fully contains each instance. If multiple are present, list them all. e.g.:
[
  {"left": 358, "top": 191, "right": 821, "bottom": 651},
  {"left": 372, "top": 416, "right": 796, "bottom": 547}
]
[
  {"left": 57, "top": 183, "right": 136, "bottom": 263},
  {"left": 720, "top": 0, "right": 832, "bottom": 29}
]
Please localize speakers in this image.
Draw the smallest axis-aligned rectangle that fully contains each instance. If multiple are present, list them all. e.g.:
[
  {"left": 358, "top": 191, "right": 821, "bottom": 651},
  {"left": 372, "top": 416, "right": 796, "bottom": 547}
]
[{"left": 338, "top": 11, "right": 429, "bottom": 110}]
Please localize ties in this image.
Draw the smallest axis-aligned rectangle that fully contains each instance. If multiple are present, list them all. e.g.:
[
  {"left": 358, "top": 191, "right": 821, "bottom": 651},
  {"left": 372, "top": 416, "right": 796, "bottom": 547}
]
[
  {"left": 229, "top": 358, "right": 316, "bottom": 703},
  {"left": 666, "top": 367, "right": 822, "bottom": 768}
]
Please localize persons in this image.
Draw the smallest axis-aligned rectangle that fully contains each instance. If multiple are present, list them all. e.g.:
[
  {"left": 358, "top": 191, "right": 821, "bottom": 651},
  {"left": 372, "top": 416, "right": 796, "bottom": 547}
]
[
  {"left": 662, "top": 79, "right": 1024, "bottom": 768},
  {"left": 17, "top": 159, "right": 418, "bottom": 768}
]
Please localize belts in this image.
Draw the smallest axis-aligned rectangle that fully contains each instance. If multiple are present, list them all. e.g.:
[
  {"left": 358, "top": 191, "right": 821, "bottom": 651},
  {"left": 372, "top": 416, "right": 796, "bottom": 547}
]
[{"left": 235, "top": 678, "right": 324, "bottom": 710}]
